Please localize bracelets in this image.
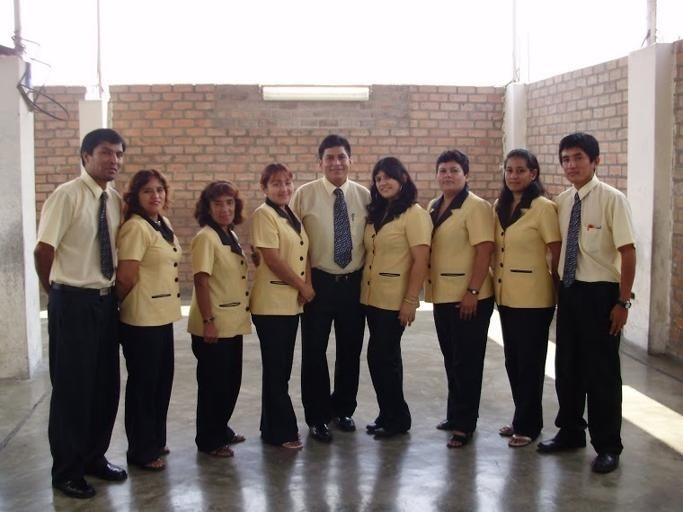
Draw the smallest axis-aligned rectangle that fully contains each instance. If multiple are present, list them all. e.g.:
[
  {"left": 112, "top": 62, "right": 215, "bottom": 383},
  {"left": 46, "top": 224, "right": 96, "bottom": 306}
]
[{"left": 402, "top": 296, "right": 420, "bottom": 307}]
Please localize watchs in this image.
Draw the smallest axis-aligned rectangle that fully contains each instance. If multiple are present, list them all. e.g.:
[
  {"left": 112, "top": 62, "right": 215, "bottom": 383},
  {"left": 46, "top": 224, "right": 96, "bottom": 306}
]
[
  {"left": 617, "top": 300, "right": 631, "bottom": 309},
  {"left": 204, "top": 315, "right": 214, "bottom": 325},
  {"left": 467, "top": 287, "right": 479, "bottom": 295}
]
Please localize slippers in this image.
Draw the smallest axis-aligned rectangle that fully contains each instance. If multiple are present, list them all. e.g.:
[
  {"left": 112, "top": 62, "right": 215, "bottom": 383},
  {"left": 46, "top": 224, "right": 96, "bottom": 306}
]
[
  {"left": 127, "top": 454, "right": 168, "bottom": 472},
  {"left": 281, "top": 438, "right": 305, "bottom": 453},
  {"left": 160, "top": 446, "right": 170, "bottom": 456}
]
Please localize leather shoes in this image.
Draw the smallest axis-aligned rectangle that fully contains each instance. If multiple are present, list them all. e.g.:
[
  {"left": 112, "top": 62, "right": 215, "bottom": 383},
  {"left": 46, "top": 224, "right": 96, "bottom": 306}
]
[
  {"left": 536, "top": 437, "right": 590, "bottom": 457},
  {"left": 309, "top": 422, "right": 333, "bottom": 444},
  {"left": 88, "top": 461, "right": 128, "bottom": 484},
  {"left": 589, "top": 445, "right": 621, "bottom": 476},
  {"left": 50, "top": 476, "right": 97, "bottom": 500},
  {"left": 332, "top": 414, "right": 357, "bottom": 434},
  {"left": 373, "top": 423, "right": 411, "bottom": 438},
  {"left": 365, "top": 419, "right": 384, "bottom": 431}
]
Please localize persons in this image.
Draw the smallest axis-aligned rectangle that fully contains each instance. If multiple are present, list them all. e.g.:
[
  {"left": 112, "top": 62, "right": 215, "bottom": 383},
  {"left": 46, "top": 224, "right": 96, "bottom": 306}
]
[
  {"left": 250, "top": 162, "right": 315, "bottom": 450},
  {"left": 538, "top": 134, "right": 637, "bottom": 475},
  {"left": 288, "top": 135, "right": 372, "bottom": 445},
  {"left": 427, "top": 148, "right": 498, "bottom": 450},
  {"left": 116, "top": 169, "right": 182, "bottom": 470},
  {"left": 186, "top": 178, "right": 251, "bottom": 461},
  {"left": 493, "top": 149, "right": 561, "bottom": 447},
  {"left": 35, "top": 127, "right": 128, "bottom": 498},
  {"left": 358, "top": 156, "right": 433, "bottom": 439}
]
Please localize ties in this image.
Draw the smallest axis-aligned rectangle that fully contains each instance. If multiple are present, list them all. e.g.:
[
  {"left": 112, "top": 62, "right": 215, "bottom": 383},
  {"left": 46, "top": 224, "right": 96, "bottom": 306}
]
[
  {"left": 95, "top": 190, "right": 117, "bottom": 283},
  {"left": 561, "top": 191, "right": 583, "bottom": 292},
  {"left": 330, "top": 186, "right": 356, "bottom": 272}
]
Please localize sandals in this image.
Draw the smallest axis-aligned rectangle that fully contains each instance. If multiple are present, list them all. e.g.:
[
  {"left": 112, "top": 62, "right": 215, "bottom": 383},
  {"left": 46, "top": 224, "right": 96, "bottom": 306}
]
[
  {"left": 496, "top": 423, "right": 511, "bottom": 438},
  {"left": 206, "top": 444, "right": 235, "bottom": 459},
  {"left": 446, "top": 432, "right": 473, "bottom": 449},
  {"left": 505, "top": 435, "right": 533, "bottom": 450},
  {"left": 227, "top": 431, "right": 246, "bottom": 444},
  {"left": 436, "top": 419, "right": 449, "bottom": 431}
]
